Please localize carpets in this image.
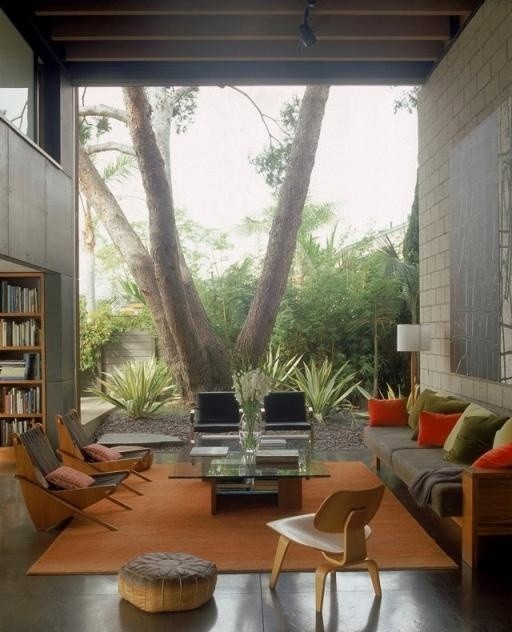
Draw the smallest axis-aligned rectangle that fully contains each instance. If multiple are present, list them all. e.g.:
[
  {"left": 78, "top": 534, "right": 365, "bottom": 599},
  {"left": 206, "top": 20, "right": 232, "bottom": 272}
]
[{"left": 26, "top": 461, "right": 460, "bottom": 576}]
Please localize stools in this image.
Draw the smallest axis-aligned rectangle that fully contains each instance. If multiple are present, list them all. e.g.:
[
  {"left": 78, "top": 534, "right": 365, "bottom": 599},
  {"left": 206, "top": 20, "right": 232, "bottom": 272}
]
[{"left": 118, "top": 552, "right": 217, "bottom": 614}]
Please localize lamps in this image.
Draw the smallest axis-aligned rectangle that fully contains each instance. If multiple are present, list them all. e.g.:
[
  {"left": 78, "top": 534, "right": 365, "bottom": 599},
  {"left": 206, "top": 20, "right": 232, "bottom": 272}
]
[{"left": 397, "top": 324, "right": 432, "bottom": 409}]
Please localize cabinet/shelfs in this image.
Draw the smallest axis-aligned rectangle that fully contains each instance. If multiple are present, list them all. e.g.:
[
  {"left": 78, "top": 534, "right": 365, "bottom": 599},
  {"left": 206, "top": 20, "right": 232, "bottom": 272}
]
[{"left": 0, "top": 272, "right": 46, "bottom": 464}]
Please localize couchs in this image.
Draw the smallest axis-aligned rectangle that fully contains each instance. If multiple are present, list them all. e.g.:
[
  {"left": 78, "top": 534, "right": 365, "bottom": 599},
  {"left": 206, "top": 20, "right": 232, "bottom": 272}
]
[{"left": 364, "top": 426, "right": 512, "bottom": 569}]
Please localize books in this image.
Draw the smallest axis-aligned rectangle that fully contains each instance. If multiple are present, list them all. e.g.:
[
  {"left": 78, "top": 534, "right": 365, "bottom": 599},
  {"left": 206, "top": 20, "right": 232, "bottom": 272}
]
[
  {"left": 259, "top": 438, "right": 287, "bottom": 447},
  {"left": 0, "top": 386, "right": 40, "bottom": 415},
  {"left": 256, "top": 449, "right": 299, "bottom": 463},
  {"left": 0, "top": 280, "right": 39, "bottom": 314},
  {"left": 0, "top": 319, "right": 41, "bottom": 347},
  {"left": 0, "top": 418, "right": 41, "bottom": 446},
  {"left": 216, "top": 478, "right": 278, "bottom": 491},
  {"left": 189, "top": 446, "right": 228, "bottom": 458},
  {"left": 0, "top": 352, "right": 40, "bottom": 380}
]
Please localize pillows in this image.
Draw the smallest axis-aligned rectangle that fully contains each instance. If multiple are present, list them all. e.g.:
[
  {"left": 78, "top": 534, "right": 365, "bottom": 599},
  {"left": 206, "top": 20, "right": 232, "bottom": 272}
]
[
  {"left": 45, "top": 466, "right": 96, "bottom": 490},
  {"left": 83, "top": 443, "right": 123, "bottom": 462},
  {"left": 367, "top": 388, "right": 512, "bottom": 482}
]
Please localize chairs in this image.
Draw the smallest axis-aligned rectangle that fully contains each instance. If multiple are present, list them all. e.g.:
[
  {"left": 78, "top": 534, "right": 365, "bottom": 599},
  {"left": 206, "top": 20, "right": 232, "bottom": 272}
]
[
  {"left": 263, "top": 391, "right": 312, "bottom": 432},
  {"left": 264, "top": 485, "right": 385, "bottom": 612},
  {"left": 13, "top": 422, "right": 143, "bottom": 532},
  {"left": 190, "top": 391, "right": 245, "bottom": 432},
  {"left": 55, "top": 408, "right": 153, "bottom": 495}
]
